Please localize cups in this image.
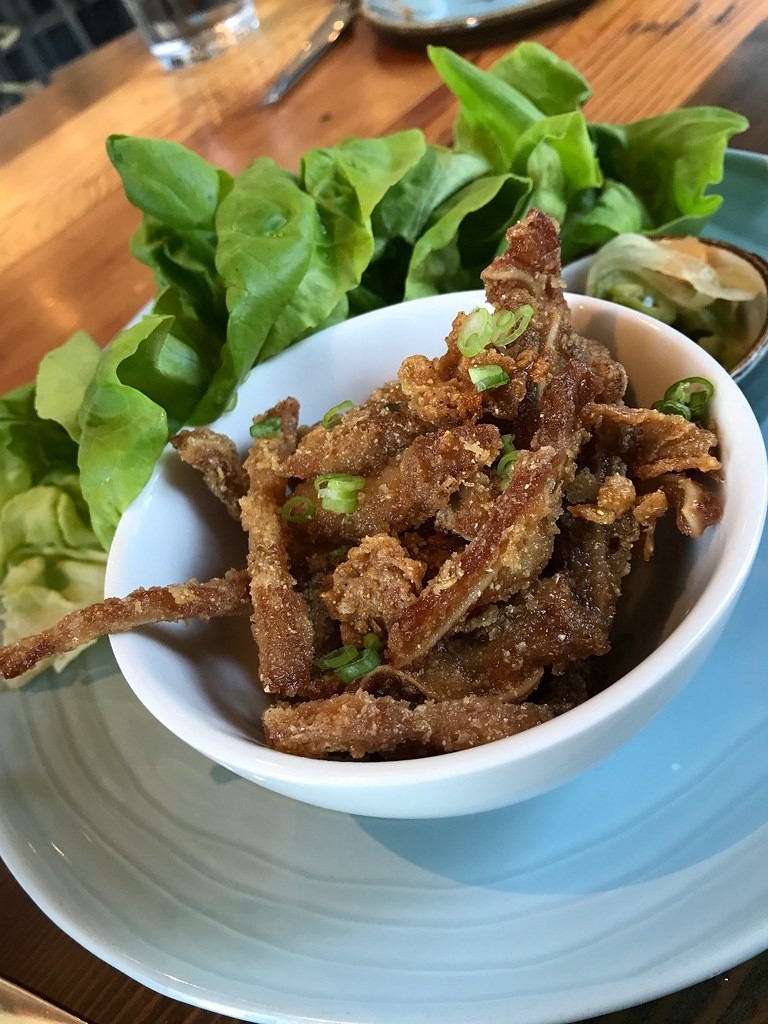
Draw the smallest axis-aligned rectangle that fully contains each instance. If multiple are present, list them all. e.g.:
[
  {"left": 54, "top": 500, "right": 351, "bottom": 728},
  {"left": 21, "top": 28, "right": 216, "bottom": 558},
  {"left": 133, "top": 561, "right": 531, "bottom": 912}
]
[{"left": 119, "top": 0, "right": 259, "bottom": 72}]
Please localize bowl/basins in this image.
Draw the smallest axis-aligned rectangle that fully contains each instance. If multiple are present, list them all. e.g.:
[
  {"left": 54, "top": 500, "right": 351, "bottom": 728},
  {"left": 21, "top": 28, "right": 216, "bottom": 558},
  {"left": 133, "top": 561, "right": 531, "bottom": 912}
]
[{"left": 104, "top": 292, "right": 768, "bottom": 819}]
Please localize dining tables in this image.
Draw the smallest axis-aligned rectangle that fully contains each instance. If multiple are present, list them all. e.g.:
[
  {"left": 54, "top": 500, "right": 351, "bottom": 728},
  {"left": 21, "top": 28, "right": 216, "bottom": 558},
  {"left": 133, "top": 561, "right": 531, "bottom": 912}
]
[{"left": 0, "top": 0, "right": 767, "bottom": 1024}]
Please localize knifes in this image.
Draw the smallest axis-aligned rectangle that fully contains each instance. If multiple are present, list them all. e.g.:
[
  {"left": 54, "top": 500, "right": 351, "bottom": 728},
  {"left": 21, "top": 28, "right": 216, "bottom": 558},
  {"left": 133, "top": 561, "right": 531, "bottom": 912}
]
[{"left": 261, "top": 0, "right": 357, "bottom": 105}]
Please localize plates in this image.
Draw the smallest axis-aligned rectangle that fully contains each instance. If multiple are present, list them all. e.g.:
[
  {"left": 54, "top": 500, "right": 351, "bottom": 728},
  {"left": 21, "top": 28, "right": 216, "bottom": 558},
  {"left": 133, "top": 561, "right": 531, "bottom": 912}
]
[
  {"left": 560, "top": 237, "right": 768, "bottom": 386},
  {"left": 360, "top": 0, "right": 576, "bottom": 42},
  {"left": 0, "top": 150, "right": 768, "bottom": 1024}
]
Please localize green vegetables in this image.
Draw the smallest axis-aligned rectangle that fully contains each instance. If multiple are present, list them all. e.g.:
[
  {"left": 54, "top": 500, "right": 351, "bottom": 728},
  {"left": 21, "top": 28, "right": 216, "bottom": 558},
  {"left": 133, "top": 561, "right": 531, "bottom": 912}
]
[{"left": 0, "top": 38, "right": 749, "bottom": 690}]
